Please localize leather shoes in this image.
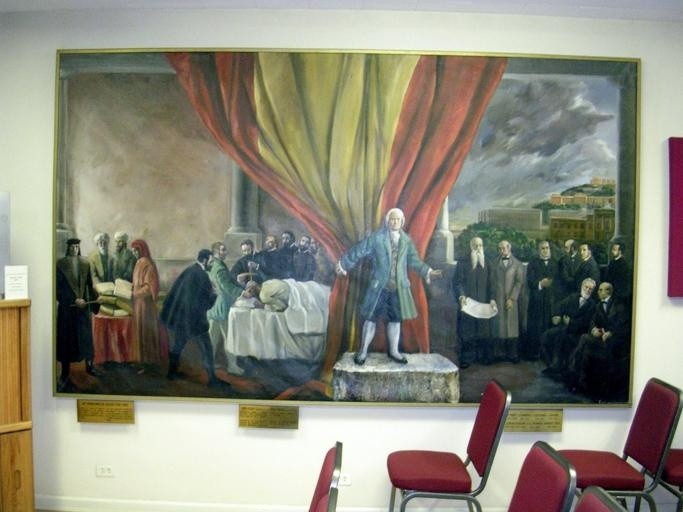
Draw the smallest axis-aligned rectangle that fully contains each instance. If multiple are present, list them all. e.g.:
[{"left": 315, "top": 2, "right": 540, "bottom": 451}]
[
  {"left": 355, "top": 351, "right": 367, "bottom": 364},
  {"left": 388, "top": 351, "right": 408, "bottom": 363},
  {"left": 461, "top": 353, "right": 540, "bottom": 369}
]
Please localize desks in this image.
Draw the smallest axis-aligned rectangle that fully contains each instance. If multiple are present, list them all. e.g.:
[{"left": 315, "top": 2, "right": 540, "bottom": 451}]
[
  {"left": 227, "top": 307, "right": 327, "bottom": 372},
  {"left": 93, "top": 311, "right": 132, "bottom": 365}
]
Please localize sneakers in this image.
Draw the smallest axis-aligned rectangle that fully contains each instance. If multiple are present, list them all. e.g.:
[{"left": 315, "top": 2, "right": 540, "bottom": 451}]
[
  {"left": 169, "top": 371, "right": 184, "bottom": 379},
  {"left": 62, "top": 378, "right": 77, "bottom": 390},
  {"left": 228, "top": 364, "right": 244, "bottom": 375},
  {"left": 209, "top": 379, "right": 230, "bottom": 387},
  {"left": 87, "top": 367, "right": 101, "bottom": 376}
]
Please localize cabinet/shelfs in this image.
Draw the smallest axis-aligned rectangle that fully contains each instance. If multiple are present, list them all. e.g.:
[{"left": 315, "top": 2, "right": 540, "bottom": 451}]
[{"left": 0, "top": 298, "right": 34, "bottom": 512}]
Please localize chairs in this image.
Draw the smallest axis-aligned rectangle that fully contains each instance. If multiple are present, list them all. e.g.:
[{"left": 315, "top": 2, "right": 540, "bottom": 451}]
[
  {"left": 388, "top": 378, "right": 683, "bottom": 512},
  {"left": 315, "top": 487, "right": 338, "bottom": 512},
  {"left": 307, "top": 441, "right": 342, "bottom": 512}
]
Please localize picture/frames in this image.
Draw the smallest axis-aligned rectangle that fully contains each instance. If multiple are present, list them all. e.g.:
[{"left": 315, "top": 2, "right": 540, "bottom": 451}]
[{"left": 51, "top": 47, "right": 641, "bottom": 409}]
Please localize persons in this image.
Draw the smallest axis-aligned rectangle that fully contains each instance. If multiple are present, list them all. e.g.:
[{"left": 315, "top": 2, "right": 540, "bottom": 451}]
[
  {"left": 335, "top": 207, "right": 445, "bottom": 366},
  {"left": 452, "top": 236, "right": 632, "bottom": 393}
]
